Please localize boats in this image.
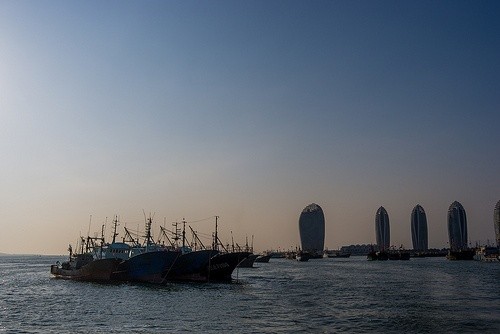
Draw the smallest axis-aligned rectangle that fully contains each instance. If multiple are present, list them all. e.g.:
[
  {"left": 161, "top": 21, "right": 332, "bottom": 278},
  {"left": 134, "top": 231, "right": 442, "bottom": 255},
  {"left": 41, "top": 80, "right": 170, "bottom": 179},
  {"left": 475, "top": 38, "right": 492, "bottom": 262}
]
[
  {"left": 50, "top": 209, "right": 274, "bottom": 285},
  {"left": 366, "top": 241, "right": 447, "bottom": 260},
  {"left": 336, "top": 246, "right": 352, "bottom": 258},
  {"left": 446, "top": 239, "right": 500, "bottom": 263},
  {"left": 263, "top": 243, "right": 311, "bottom": 262}
]
